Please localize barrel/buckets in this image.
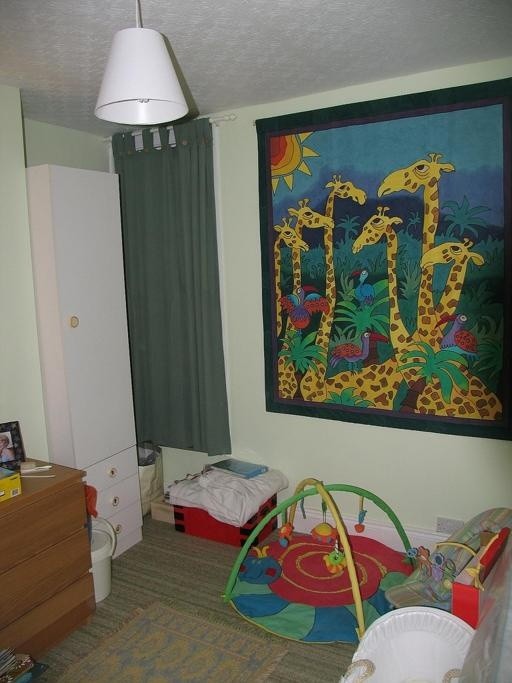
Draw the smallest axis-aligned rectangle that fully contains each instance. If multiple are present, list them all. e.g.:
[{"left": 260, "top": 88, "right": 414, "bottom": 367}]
[{"left": 87, "top": 517, "right": 117, "bottom": 604}]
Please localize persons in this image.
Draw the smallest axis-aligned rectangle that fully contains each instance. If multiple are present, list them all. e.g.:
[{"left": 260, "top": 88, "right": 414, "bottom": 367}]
[{"left": 0, "top": 434, "right": 14, "bottom": 462}]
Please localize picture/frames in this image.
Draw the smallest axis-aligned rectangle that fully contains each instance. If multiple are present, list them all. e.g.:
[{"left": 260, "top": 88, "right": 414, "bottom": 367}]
[
  {"left": 2, "top": 421, "right": 24, "bottom": 470},
  {"left": 254, "top": 76, "right": 511, "bottom": 445}
]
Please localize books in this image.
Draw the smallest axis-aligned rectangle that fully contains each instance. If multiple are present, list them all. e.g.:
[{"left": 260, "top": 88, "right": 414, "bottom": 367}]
[{"left": 0, "top": 645, "right": 47, "bottom": 683}]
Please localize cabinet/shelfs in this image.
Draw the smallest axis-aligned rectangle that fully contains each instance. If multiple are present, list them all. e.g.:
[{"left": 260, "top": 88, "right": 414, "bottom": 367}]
[
  {"left": 24, "top": 162, "right": 144, "bottom": 561},
  {"left": 0, "top": 456, "right": 98, "bottom": 665}
]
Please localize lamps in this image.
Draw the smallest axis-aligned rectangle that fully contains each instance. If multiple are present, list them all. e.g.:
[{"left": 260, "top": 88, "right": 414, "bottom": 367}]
[{"left": 93, "top": 2, "right": 189, "bottom": 130}]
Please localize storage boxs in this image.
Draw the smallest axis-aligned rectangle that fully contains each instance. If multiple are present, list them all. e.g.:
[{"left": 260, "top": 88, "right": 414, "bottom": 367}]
[
  {"left": 175, "top": 482, "right": 279, "bottom": 547},
  {"left": 152, "top": 493, "right": 174, "bottom": 526}
]
